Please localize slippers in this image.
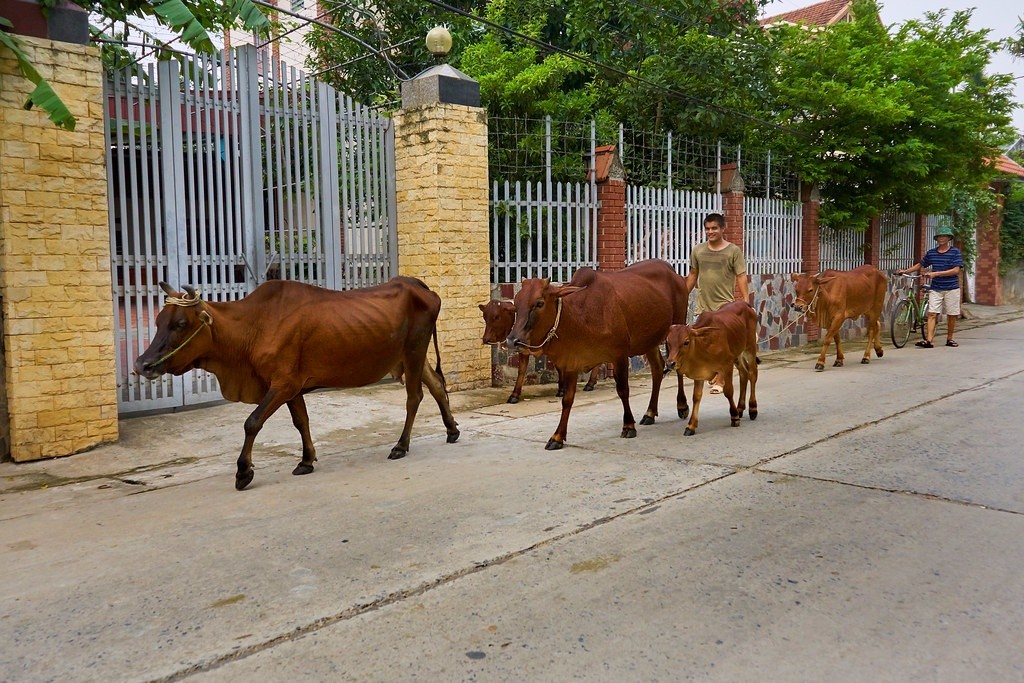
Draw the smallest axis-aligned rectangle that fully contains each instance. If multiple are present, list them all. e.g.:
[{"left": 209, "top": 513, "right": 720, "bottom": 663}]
[{"left": 708, "top": 375, "right": 724, "bottom": 394}]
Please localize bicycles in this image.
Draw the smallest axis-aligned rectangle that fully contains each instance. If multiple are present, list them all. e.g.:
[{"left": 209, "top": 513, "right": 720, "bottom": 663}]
[{"left": 890, "top": 272, "right": 938, "bottom": 349}]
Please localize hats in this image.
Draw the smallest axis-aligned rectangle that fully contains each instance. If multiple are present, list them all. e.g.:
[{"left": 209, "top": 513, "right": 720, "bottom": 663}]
[{"left": 934, "top": 228, "right": 954, "bottom": 240}]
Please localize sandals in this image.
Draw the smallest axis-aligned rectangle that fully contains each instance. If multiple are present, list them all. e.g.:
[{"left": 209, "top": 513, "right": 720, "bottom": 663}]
[
  {"left": 915, "top": 340, "right": 934, "bottom": 348},
  {"left": 946, "top": 339, "right": 958, "bottom": 347}
]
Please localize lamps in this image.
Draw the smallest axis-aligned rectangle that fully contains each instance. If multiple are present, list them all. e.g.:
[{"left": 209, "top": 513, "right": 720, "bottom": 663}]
[{"left": 425, "top": 27, "right": 452, "bottom": 65}]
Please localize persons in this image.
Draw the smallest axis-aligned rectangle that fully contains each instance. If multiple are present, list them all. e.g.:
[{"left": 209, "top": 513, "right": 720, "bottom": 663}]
[
  {"left": 895, "top": 227, "right": 964, "bottom": 348},
  {"left": 685, "top": 213, "right": 750, "bottom": 394}
]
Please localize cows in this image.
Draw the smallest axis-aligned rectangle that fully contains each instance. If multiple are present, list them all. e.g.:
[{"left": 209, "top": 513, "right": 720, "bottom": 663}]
[
  {"left": 132, "top": 275, "right": 459, "bottom": 491},
  {"left": 505, "top": 258, "right": 689, "bottom": 450},
  {"left": 665, "top": 300, "right": 761, "bottom": 437},
  {"left": 477, "top": 298, "right": 601, "bottom": 405},
  {"left": 790, "top": 264, "right": 887, "bottom": 371}
]
